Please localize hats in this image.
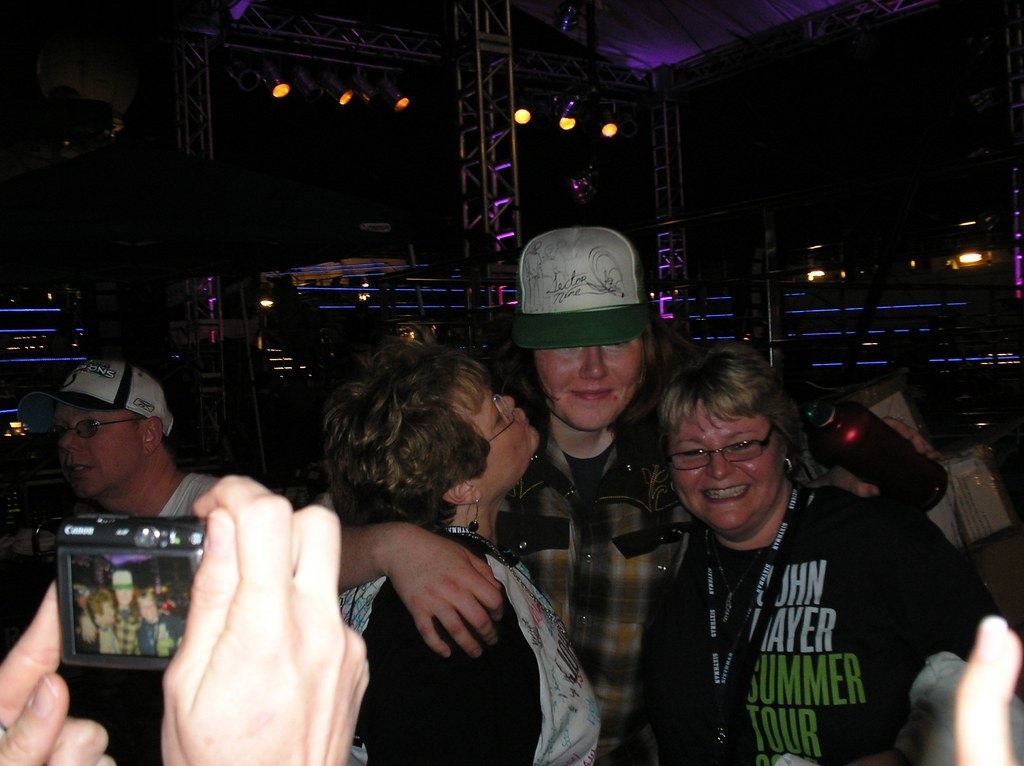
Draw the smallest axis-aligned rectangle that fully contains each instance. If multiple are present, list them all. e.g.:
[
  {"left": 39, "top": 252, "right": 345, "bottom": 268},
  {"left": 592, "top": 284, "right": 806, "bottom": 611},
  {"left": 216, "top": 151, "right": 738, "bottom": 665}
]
[
  {"left": 513, "top": 225, "right": 648, "bottom": 350},
  {"left": 19, "top": 359, "right": 175, "bottom": 437},
  {"left": 112, "top": 569, "right": 133, "bottom": 591}
]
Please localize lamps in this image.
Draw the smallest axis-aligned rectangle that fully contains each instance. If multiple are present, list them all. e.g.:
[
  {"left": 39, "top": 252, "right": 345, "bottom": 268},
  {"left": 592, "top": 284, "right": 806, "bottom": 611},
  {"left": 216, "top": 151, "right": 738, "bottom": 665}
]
[
  {"left": 320, "top": 72, "right": 354, "bottom": 105},
  {"left": 601, "top": 110, "right": 618, "bottom": 137},
  {"left": 556, "top": 89, "right": 582, "bottom": 117},
  {"left": 263, "top": 65, "right": 291, "bottom": 98},
  {"left": 381, "top": 79, "right": 409, "bottom": 112},
  {"left": 292, "top": 69, "right": 321, "bottom": 101},
  {"left": 553, "top": 2, "right": 580, "bottom": 31},
  {"left": 351, "top": 74, "right": 374, "bottom": 101}
]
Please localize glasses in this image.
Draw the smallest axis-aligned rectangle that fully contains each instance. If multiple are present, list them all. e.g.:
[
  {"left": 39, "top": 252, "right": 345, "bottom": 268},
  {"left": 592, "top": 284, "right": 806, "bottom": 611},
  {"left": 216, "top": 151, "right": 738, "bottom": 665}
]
[
  {"left": 487, "top": 393, "right": 515, "bottom": 441},
  {"left": 49, "top": 418, "right": 139, "bottom": 438},
  {"left": 667, "top": 423, "right": 774, "bottom": 471}
]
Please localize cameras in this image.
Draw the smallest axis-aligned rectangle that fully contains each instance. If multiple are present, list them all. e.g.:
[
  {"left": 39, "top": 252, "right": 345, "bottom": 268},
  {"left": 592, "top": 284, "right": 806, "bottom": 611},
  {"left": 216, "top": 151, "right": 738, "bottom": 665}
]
[{"left": 56, "top": 510, "right": 207, "bottom": 670}]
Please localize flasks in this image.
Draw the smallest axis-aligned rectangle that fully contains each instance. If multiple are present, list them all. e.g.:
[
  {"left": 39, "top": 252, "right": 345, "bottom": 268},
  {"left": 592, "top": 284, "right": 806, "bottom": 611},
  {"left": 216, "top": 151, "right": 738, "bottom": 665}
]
[{"left": 807, "top": 399, "right": 948, "bottom": 515}]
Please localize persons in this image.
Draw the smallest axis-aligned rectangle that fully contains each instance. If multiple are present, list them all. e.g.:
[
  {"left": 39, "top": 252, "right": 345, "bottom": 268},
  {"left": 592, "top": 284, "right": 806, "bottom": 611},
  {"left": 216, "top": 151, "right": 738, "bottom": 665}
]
[
  {"left": 0, "top": 228, "right": 1023, "bottom": 766},
  {"left": 135, "top": 593, "right": 185, "bottom": 658},
  {"left": 74, "top": 588, "right": 121, "bottom": 656},
  {"left": 78, "top": 569, "right": 170, "bottom": 655}
]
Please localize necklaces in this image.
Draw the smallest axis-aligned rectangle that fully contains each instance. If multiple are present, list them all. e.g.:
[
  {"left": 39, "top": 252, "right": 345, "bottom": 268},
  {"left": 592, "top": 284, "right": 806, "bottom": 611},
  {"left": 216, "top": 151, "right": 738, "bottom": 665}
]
[{"left": 482, "top": 540, "right": 569, "bottom": 636}]
